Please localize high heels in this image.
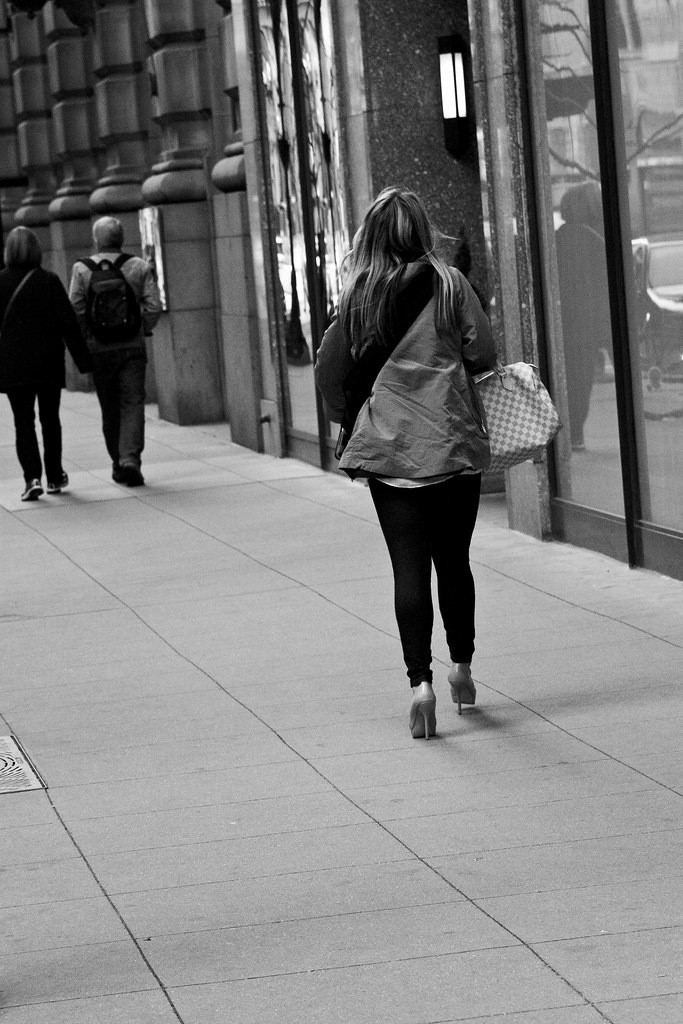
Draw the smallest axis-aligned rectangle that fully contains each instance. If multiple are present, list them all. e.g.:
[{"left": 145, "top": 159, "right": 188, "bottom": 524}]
[
  {"left": 448, "top": 663, "right": 477, "bottom": 715},
  {"left": 409, "top": 682, "right": 437, "bottom": 740}
]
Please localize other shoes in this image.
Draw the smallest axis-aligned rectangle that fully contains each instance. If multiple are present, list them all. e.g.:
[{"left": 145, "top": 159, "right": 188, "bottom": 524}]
[
  {"left": 122, "top": 468, "right": 146, "bottom": 487},
  {"left": 111, "top": 462, "right": 126, "bottom": 485}
]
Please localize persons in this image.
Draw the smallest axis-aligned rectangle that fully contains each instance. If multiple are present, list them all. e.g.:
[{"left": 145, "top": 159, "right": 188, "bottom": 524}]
[
  {"left": 67, "top": 215, "right": 162, "bottom": 487},
  {"left": 311, "top": 188, "right": 496, "bottom": 738},
  {"left": 551, "top": 182, "right": 612, "bottom": 450},
  {"left": 0, "top": 226, "right": 90, "bottom": 501}
]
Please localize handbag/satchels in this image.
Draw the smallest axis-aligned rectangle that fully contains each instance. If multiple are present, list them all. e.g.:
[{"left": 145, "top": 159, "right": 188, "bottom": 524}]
[{"left": 472, "top": 359, "right": 563, "bottom": 475}]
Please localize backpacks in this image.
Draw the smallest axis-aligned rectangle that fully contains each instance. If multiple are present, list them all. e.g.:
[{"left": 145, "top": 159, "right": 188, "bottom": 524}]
[{"left": 80, "top": 255, "right": 142, "bottom": 346}]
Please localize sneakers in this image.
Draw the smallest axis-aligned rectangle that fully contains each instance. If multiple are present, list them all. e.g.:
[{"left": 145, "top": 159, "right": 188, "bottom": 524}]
[
  {"left": 20, "top": 479, "right": 44, "bottom": 501},
  {"left": 46, "top": 470, "right": 69, "bottom": 494}
]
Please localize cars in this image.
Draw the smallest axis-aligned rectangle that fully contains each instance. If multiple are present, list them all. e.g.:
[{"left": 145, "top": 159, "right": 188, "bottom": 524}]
[{"left": 621, "top": 155, "right": 683, "bottom": 386}]
